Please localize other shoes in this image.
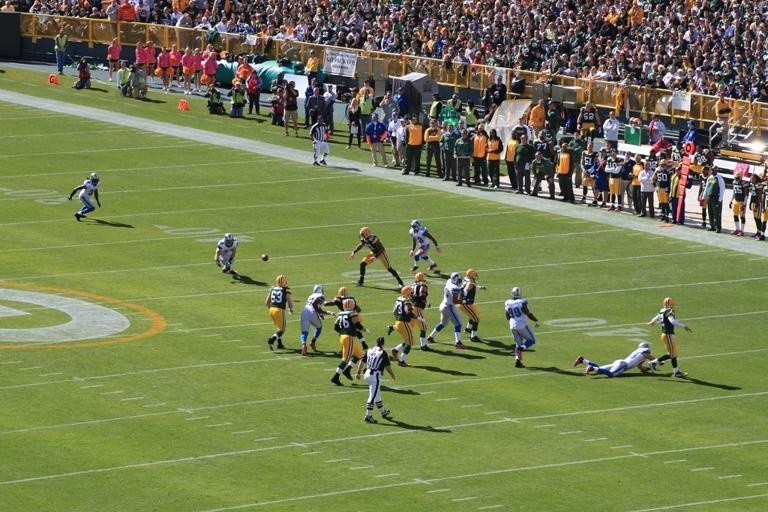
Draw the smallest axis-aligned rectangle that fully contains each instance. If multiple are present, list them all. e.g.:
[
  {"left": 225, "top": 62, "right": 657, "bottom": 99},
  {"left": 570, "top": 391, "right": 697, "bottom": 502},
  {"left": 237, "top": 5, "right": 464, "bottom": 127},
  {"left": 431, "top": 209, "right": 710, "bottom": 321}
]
[
  {"left": 313, "top": 160, "right": 326, "bottom": 166},
  {"left": 373, "top": 159, "right": 444, "bottom": 178},
  {"left": 74, "top": 213, "right": 86, "bottom": 222},
  {"left": 443, "top": 176, "right": 585, "bottom": 204},
  {"left": 588, "top": 200, "right": 685, "bottom": 225}
]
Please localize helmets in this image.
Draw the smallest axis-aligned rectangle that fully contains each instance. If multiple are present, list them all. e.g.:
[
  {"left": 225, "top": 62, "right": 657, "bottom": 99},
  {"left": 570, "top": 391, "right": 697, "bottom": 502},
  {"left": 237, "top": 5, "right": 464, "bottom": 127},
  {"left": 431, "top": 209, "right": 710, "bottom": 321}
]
[
  {"left": 359, "top": 219, "right": 423, "bottom": 236},
  {"left": 450, "top": 268, "right": 479, "bottom": 283},
  {"left": 224, "top": 232, "right": 233, "bottom": 242},
  {"left": 511, "top": 287, "right": 522, "bottom": 299},
  {"left": 276, "top": 274, "right": 355, "bottom": 310},
  {"left": 638, "top": 342, "right": 651, "bottom": 354},
  {"left": 90, "top": 173, "right": 99, "bottom": 184},
  {"left": 662, "top": 297, "right": 674, "bottom": 308},
  {"left": 398, "top": 273, "right": 425, "bottom": 305}
]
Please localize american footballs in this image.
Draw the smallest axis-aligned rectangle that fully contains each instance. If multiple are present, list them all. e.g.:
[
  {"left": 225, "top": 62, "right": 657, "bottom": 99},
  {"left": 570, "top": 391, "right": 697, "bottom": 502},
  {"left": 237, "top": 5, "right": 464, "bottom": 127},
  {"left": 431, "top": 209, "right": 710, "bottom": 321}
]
[{"left": 261, "top": 254, "right": 268, "bottom": 261}]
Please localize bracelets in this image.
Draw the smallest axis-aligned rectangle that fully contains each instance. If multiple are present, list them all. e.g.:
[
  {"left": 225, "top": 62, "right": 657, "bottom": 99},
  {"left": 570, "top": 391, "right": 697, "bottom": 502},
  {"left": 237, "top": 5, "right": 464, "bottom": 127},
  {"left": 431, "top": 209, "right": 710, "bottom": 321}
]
[
  {"left": 535, "top": 321, "right": 539, "bottom": 325},
  {"left": 462, "top": 299, "right": 466, "bottom": 304}
]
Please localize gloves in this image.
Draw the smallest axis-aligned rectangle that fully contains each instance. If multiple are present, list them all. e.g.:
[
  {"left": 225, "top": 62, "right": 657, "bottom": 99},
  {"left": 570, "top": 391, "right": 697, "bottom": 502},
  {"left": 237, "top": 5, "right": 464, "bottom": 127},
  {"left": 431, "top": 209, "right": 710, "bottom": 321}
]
[
  {"left": 462, "top": 284, "right": 495, "bottom": 304},
  {"left": 288, "top": 308, "right": 294, "bottom": 315},
  {"left": 331, "top": 312, "right": 336, "bottom": 316},
  {"left": 534, "top": 320, "right": 540, "bottom": 329},
  {"left": 347, "top": 247, "right": 446, "bottom": 261}
]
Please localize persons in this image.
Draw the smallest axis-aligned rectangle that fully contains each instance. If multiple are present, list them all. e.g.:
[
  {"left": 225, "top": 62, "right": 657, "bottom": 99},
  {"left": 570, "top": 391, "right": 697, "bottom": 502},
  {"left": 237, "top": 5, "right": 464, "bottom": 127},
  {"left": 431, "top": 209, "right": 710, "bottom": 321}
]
[
  {"left": 388, "top": 273, "right": 433, "bottom": 351},
  {"left": 356, "top": 337, "right": 395, "bottom": 423},
  {"left": 648, "top": 297, "right": 693, "bottom": 377},
  {"left": 350, "top": 227, "right": 404, "bottom": 288},
  {"left": 457, "top": 269, "right": 486, "bottom": 342},
  {"left": 505, "top": 287, "right": 539, "bottom": 367},
  {"left": 574, "top": 342, "right": 664, "bottom": 377},
  {"left": 301, "top": 285, "right": 336, "bottom": 355},
  {"left": 331, "top": 299, "right": 370, "bottom": 386},
  {"left": 409, "top": 220, "right": 441, "bottom": 271},
  {"left": 266, "top": 276, "right": 293, "bottom": 351},
  {"left": 427, "top": 273, "right": 467, "bottom": 348},
  {"left": 392, "top": 285, "right": 425, "bottom": 366},
  {"left": 324, "top": 287, "right": 368, "bottom": 355},
  {"left": 215, "top": 233, "right": 238, "bottom": 274}
]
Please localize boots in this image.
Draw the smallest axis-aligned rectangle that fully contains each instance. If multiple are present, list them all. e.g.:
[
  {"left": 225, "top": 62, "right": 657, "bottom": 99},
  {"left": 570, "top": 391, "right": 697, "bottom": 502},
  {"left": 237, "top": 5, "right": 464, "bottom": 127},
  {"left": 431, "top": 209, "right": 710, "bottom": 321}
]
[
  {"left": 267, "top": 337, "right": 288, "bottom": 352},
  {"left": 331, "top": 364, "right": 360, "bottom": 386}
]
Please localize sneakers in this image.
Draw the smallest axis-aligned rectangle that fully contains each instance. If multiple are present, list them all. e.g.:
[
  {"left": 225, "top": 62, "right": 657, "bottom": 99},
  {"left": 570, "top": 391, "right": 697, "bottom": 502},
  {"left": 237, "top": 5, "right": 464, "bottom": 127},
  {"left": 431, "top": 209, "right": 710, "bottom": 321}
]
[
  {"left": 708, "top": 227, "right": 766, "bottom": 239},
  {"left": 648, "top": 361, "right": 694, "bottom": 375},
  {"left": 573, "top": 355, "right": 594, "bottom": 375},
  {"left": 381, "top": 408, "right": 390, "bottom": 419},
  {"left": 221, "top": 267, "right": 239, "bottom": 273},
  {"left": 408, "top": 262, "right": 437, "bottom": 272},
  {"left": 351, "top": 280, "right": 364, "bottom": 287},
  {"left": 365, "top": 415, "right": 378, "bottom": 424},
  {"left": 382, "top": 325, "right": 483, "bottom": 367},
  {"left": 301, "top": 341, "right": 317, "bottom": 355},
  {"left": 514, "top": 343, "right": 526, "bottom": 368}
]
